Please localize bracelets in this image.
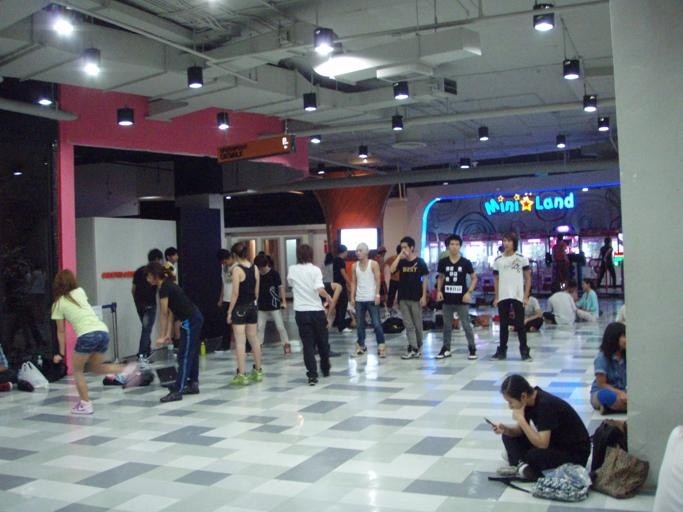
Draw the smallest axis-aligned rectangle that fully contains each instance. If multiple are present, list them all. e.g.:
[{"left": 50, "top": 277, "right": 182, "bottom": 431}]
[{"left": 59, "top": 353, "right": 64, "bottom": 358}]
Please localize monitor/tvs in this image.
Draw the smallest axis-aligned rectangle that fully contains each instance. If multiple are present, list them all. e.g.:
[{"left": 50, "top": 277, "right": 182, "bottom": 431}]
[{"left": 337, "top": 227, "right": 381, "bottom": 254}]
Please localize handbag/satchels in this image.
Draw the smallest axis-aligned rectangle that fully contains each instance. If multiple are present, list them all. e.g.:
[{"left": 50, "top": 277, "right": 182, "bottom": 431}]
[
  {"left": 591, "top": 445, "right": 649, "bottom": 500},
  {"left": 534, "top": 463, "right": 591, "bottom": 503},
  {"left": 17, "top": 361, "right": 49, "bottom": 389}
]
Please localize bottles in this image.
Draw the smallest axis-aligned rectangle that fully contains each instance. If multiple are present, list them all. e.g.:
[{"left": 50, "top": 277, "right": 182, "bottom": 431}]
[
  {"left": 138, "top": 354, "right": 145, "bottom": 369},
  {"left": 200, "top": 340, "right": 207, "bottom": 356},
  {"left": 36, "top": 355, "right": 43, "bottom": 366}
]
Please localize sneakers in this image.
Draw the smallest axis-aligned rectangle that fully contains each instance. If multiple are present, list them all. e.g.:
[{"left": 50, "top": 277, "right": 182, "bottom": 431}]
[
  {"left": 69, "top": 399, "right": 95, "bottom": 416},
  {"left": 158, "top": 391, "right": 184, "bottom": 403},
  {"left": 527, "top": 315, "right": 555, "bottom": 333},
  {"left": 226, "top": 368, "right": 252, "bottom": 387},
  {"left": 248, "top": 362, "right": 265, "bottom": 384},
  {"left": 180, "top": 381, "right": 201, "bottom": 394},
  {"left": 516, "top": 462, "right": 535, "bottom": 480},
  {"left": 0, "top": 382, "right": 13, "bottom": 391},
  {"left": 279, "top": 343, "right": 341, "bottom": 385},
  {"left": 375, "top": 342, "right": 389, "bottom": 357},
  {"left": 399, "top": 344, "right": 535, "bottom": 362},
  {"left": 350, "top": 342, "right": 369, "bottom": 357}
]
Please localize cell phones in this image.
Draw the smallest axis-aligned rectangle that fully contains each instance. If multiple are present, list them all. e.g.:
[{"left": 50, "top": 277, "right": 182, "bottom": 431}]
[{"left": 484, "top": 417, "right": 495, "bottom": 427}]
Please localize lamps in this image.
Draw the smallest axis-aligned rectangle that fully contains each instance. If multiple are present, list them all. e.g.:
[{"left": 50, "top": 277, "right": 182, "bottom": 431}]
[
  {"left": 596, "top": 115, "right": 612, "bottom": 136},
  {"left": 531, "top": 1, "right": 556, "bottom": 34},
  {"left": 187, "top": 29, "right": 203, "bottom": 90},
  {"left": 216, "top": 104, "right": 231, "bottom": 130},
  {"left": 394, "top": 82, "right": 409, "bottom": 100},
  {"left": 36, "top": 84, "right": 57, "bottom": 108},
  {"left": 561, "top": 53, "right": 582, "bottom": 82},
  {"left": 477, "top": 120, "right": 490, "bottom": 144},
  {"left": 116, "top": 94, "right": 135, "bottom": 125},
  {"left": 390, "top": 111, "right": 405, "bottom": 134},
  {"left": 301, "top": 74, "right": 318, "bottom": 111},
  {"left": 581, "top": 86, "right": 600, "bottom": 113},
  {"left": 313, "top": 27, "right": 343, "bottom": 56},
  {"left": 81, "top": 21, "right": 101, "bottom": 74},
  {"left": 306, "top": 136, "right": 472, "bottom": 178},
  {"left": 555, "top": 130, "right": 569, "bottom": 153}
]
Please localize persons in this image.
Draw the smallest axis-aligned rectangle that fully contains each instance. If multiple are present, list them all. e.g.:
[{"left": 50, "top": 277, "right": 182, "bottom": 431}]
[
  {"left": 382, "top": 234, "right": 534, "bottom": 361},
  {"left": 590, "top": 322, "right": 627, "bottom": 415},
  {"left": 132, "top": 248, "right": 164, "bottom": 359},
  {"left": 491, "top": 375, "right": 590, "bottom": 482},
  {"left": 51, "top": 268, "right": 142, "bottom": 415},
  {"left": 164, "top": 247, "right": 181, "bottom": 350},
  {"left": 217, "top": 234, "right": 386, "bottom": 385},
  {"left": 508, "top": 234, "right": 617, "bottom": 333},
  {"left": 143, "top": 262, "right": 204, "bottom": 402},
  {"left": 3, "top": 264, "right": 49, "bottom": 350}
]
